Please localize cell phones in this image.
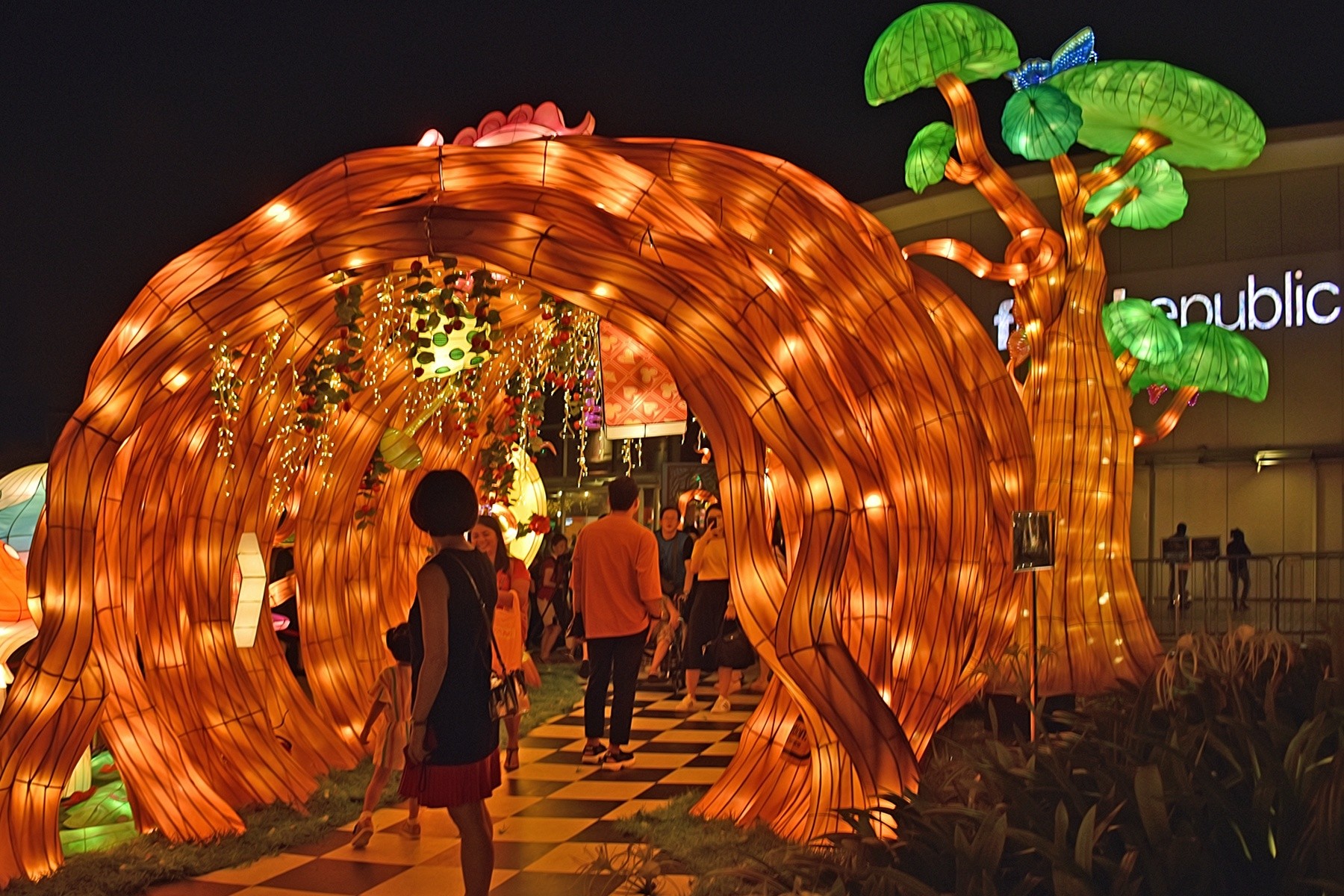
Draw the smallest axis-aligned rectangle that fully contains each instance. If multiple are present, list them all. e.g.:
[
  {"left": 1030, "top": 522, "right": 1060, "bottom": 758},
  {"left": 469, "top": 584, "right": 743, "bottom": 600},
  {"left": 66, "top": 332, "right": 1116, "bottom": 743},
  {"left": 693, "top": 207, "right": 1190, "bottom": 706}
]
[{"left": 707, "top": 517, "right": 717, "bottom": 530}]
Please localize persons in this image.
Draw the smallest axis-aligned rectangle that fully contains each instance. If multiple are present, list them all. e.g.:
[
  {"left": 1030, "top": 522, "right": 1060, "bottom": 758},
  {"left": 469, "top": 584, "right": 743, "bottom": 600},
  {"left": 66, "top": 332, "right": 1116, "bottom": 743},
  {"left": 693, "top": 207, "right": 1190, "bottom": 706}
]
[
  {"left": 570, "top": 476, "right": 664, "bottom": 770},
  {"left": 532, "top": 503, "right": 770, "bottom": 712},
  {"left": 352, "top": 623, "right": 422, "bottom": 847},
  {"left": 466, "top": 516, "right": 531, "bottom": 774},
  {"left": 399, "top": 469, "right": 501, "bottom": 896},
  {"left": 1165, "top": 523, "right": 1192, "bottom": 609},
  {"left": 1227, "top": 527, "right": 1253, "bottom": 612}
]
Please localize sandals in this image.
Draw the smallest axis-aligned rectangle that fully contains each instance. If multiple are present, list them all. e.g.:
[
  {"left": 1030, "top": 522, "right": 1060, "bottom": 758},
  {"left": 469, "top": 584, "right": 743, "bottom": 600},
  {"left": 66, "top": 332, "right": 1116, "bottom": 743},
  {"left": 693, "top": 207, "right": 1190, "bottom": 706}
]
[{"left": 504, "top": 747, "right": 519, "bottom": 771}]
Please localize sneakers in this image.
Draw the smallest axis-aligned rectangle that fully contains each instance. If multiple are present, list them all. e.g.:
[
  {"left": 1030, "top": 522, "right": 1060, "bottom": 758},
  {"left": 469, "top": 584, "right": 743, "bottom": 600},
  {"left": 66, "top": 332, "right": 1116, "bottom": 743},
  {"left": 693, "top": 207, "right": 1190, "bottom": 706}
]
[
  {"left": 675, "top": 694, "right": 696, "bottom": 709},
  {"left": 582, "top": 743, "right": 609, "bottom": 763},
  {"left": 603, "top": 750, "right": 636, "bottom": 770},
  {"left": 711, "top": 697, "right": 731, "bottom": 712}
]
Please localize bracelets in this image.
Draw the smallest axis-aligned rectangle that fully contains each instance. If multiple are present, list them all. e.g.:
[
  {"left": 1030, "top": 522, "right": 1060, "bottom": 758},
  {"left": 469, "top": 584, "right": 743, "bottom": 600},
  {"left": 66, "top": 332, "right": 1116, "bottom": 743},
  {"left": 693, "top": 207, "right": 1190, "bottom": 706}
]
[
  {"left": 727, "top": 601, "right": 735, "bottom": 605},
  {"left": 650, "top": 615, "right": 662, "bottom": 620},
  {"left": 410, "top": 718, "right": 427, "bottom": 727}
]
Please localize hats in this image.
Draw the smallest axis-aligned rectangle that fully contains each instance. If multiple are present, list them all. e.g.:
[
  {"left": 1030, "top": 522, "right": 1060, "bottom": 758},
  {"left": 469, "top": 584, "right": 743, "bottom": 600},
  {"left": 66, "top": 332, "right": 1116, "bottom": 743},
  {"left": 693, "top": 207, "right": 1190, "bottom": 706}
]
[{"left": 385, "top": 623, "right": 411, "bottom": 662}]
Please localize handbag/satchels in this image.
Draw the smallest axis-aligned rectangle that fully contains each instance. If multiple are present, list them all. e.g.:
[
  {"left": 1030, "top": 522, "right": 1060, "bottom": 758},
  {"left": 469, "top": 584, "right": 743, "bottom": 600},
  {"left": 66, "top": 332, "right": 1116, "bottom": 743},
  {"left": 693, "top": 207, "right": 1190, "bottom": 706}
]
[
  {"left": 566, "top": 611, "right": 584, "bottom": 638},
  {"left": 491, "top": 669, "right": 530, "bottom": 720},
  {"left": 717, "top": 616, "right": 756, "bottom": 668},
  {"left": 523, "top": 653, "right": 541, "bottom": 687},
  {"left": 492, "top": 589, "right": 521, "bottom": 673}
]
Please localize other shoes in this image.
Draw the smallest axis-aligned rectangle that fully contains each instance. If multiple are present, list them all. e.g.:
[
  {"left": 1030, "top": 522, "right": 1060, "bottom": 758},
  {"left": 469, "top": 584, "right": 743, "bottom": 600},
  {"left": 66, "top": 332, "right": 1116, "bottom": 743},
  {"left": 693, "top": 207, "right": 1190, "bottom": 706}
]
[
  {"left": 579, "top": 660, "right": 589, "bottom": 679},
  {"left": 400, "top": 821, "right": 418, "bottom": 839},
  {"left": 351, "top": 817, "right": 373, "bottom": 847},
  {"left": 648, "top": 671, "right": 658, "bottom": 679}
]
